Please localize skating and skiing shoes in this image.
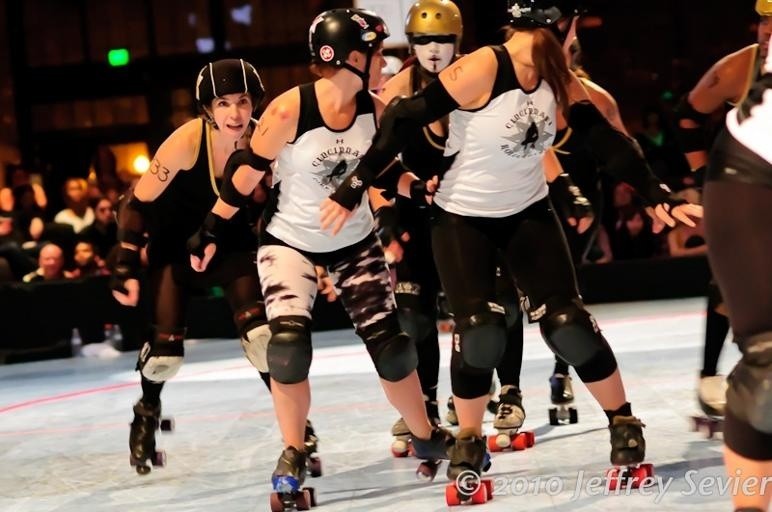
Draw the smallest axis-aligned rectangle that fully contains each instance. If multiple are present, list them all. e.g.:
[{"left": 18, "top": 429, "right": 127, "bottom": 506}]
[
  {"left": 689, "top": 375, "right": 730, "bottom": 436},
  {"left": 391, "top": 385, "right": 535, "bottom": 507},
  {"left": 549, "top": 375, "right": 576, "bottom": 424},
  {"left": 606, "top": 418, "right": 654, "bottom": 489},
  {"left": 129, "top": 402, "right": 173, "bottom": 475},
  {"left": 270, "top": 421, "right": 323, "bottom": 511}
]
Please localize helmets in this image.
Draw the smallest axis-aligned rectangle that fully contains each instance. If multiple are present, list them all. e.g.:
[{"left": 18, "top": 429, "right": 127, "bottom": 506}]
[
  {"left": 309, "top": 9, "right": 390, "bottom": 68},
  {"left": 756, "top": 1, "right": 771, "bottom": 16},
  {"left": 504, "top": 1, "right": 585, "bottom": 31},
  {"left": 406, "top": 1, "right": 463, "bottom": 34},
  {"left": 195, "top": 58, "right": 265, "bottom": 102}
]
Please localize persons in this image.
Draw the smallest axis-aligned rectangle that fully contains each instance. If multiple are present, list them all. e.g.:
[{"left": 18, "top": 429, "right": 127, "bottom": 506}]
[
  {"left": 112, "top": 57, "right": 321, "bottom": 479},
  {"left": 676, "top": 1, "right": 771, "bottom": 418},
  {"left": 1, "top": 168, "right": 717, "bottom": 365},
  {"left": 317, "top": 1, "right": 704, "bottom": 481},
  {"left": 364, "top": 0, "right": 596, "bottom": 440},
  {"left": 699, "top": 31, "right": 770, "bottom": 511},
  {"left": 187, "top": 7, "right": 458, "bottom": 499},
  {"left": 492, "top": 16, "right": 666, "bottom": 429}
]
